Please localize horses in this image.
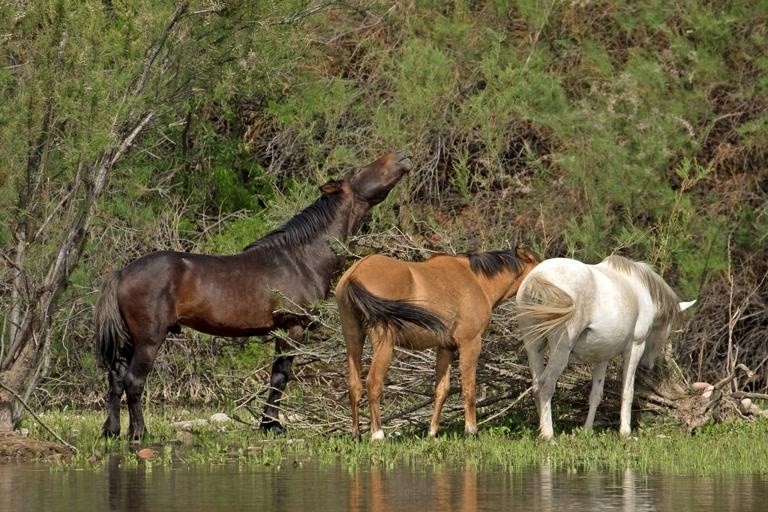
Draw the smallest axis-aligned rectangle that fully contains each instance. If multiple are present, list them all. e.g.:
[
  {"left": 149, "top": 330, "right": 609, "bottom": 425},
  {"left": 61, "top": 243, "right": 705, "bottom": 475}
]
[
  {"left": 504, "top": 254, "right": 696, "bottom": 440},
  {"left": 336, "top": 246, "right": 541, "bottom": 444},
  {"left": 94, "top": 147, "right": 417, "bottom": 435}
]
